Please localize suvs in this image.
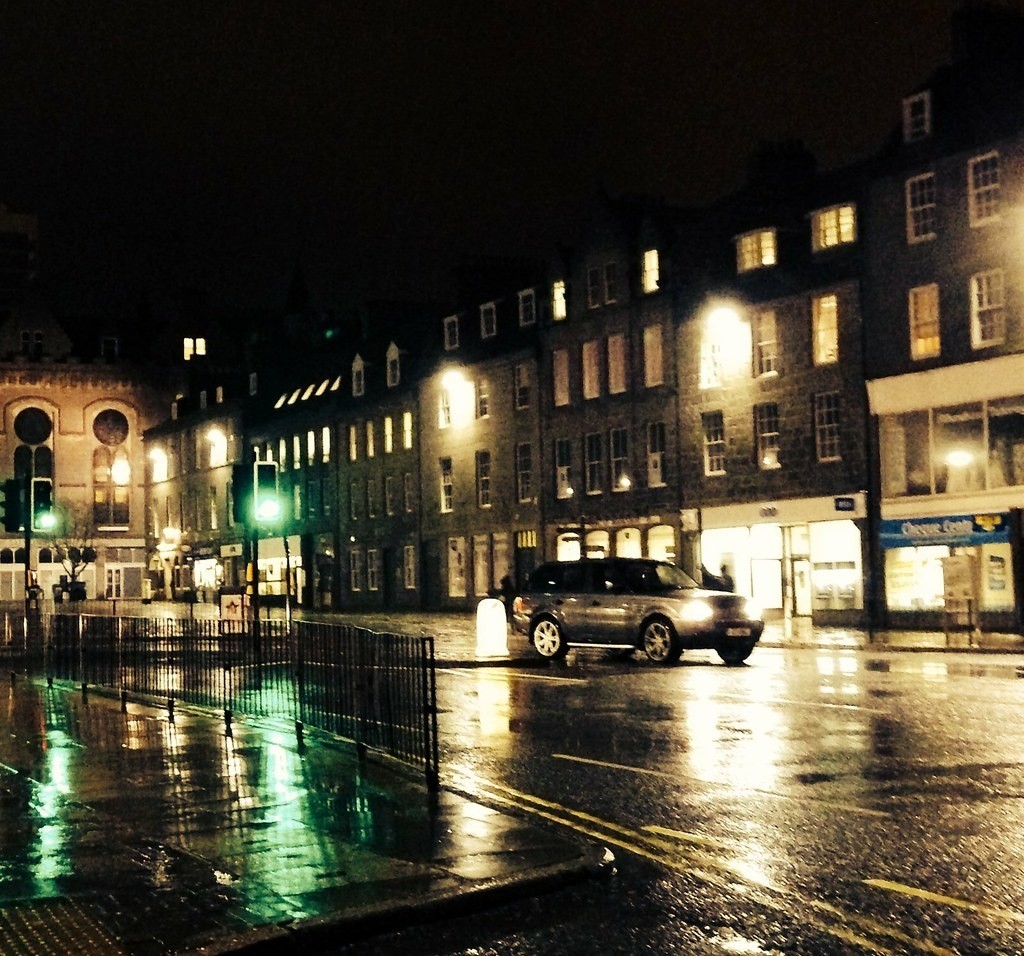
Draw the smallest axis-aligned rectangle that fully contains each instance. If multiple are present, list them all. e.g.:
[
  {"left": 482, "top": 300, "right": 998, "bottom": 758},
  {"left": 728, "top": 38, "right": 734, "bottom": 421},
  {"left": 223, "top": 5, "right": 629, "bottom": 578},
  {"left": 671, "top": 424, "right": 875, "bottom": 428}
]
[{"left": 513, "top": 558, "right": 765, "bottom": 668}]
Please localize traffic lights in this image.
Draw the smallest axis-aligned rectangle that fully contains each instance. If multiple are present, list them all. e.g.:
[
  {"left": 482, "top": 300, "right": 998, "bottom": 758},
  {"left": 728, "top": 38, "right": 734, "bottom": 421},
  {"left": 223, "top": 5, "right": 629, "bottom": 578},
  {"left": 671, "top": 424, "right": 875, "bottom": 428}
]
[
  {"left": 0, "top": 481, "right": 25, "bottom": 532},
  {"left": 254, "top": 461, "right": 281, "bottom": 524},
  {"left": 29, "top": 477, "right": 56, "bottom": 534}
]
